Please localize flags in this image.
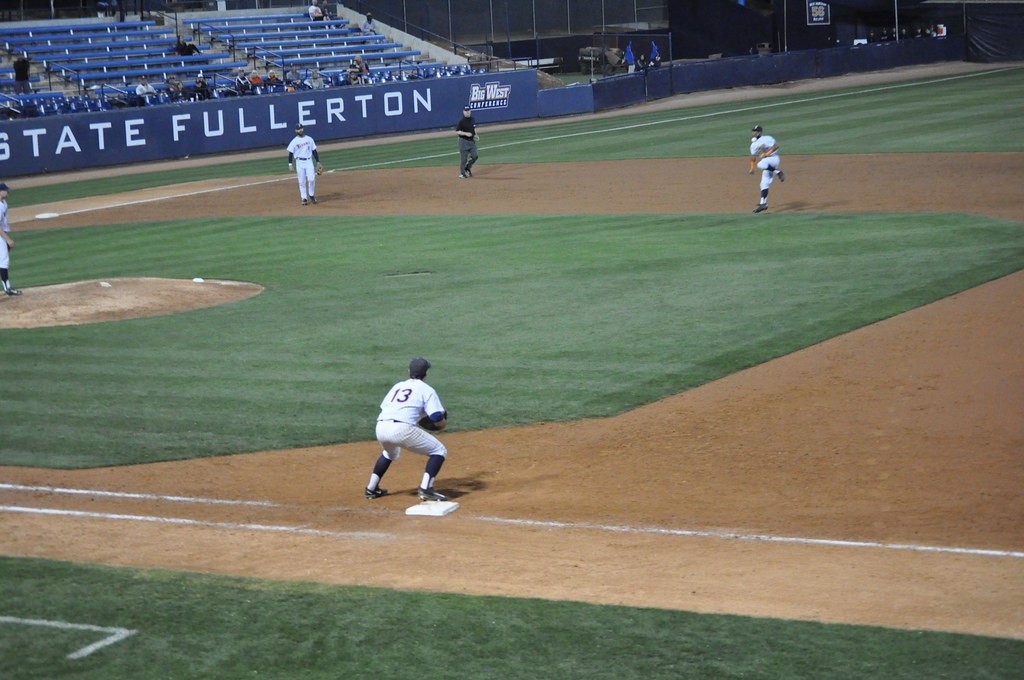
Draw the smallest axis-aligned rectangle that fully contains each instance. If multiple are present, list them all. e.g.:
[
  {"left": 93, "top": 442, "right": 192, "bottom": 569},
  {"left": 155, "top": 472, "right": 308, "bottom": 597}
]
[
  {"left": 621, "top": 45, "right": 635, "bottom": 74},
  {"left": 640, "top": 42, "right": 661, "bottom": 68}
]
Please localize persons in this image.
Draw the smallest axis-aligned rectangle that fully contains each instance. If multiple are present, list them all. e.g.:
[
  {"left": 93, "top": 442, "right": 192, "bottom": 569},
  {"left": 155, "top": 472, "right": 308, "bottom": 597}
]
[
  {"left": 749, "top": 124, "right": 785, "bottom": 213},
  {"left": 135, "top": 55, "right": 369, "bottom": 107},
  {"left": 13, "top": 49, "right": 31, "bottom": 94},
  {"left": 309, "top": 0, "right": 336, "bottom": 30},
  {"left": 456, "top": 104, "right": 479, "bottom": 179},
  {"left": 0, "top": 182, "right": 24, "bottom": 296},
  {"left": 174, "top": 35, "right": 202, "bottom": 57},
  {"left": 286, "top": 123, "right": 322, "bottom": 206},
  {"left": 360, "top": 12, "right": 379, "bottom": 43},
  {"left": 364, "top": 357, "right": 449, "bottom": 501}
]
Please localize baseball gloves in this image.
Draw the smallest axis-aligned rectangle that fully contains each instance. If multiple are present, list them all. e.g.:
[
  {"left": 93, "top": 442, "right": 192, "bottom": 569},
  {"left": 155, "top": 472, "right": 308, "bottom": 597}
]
[
  {"left": 316, "top": 162, "right": 323, "bottom": 176},
  {"left": 751, "top": 153, "right": 762, "bottom": 163},
  {"left": 419, "top": 411, "right": 447, "bottom": 431}
]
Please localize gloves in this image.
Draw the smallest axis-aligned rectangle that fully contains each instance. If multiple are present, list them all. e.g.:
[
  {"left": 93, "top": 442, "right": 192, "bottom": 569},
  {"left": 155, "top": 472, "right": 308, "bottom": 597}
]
[{"left": 288, "top": 163, "right": 295, "bottom": 173}]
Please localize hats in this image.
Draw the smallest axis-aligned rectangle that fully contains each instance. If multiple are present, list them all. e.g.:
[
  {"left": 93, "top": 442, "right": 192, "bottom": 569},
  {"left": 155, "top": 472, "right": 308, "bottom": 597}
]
[
  {"left": 295, "top": 124, "right": 303, "bottom": 129},
  {"left": 409, "top": 357, "right": 431, "bottom": 376},
  {"left": 462, "top": 105, "right": 471, "bottom": 111},
  {"left": 141, "top": 75, "right": 147, "bottom": 80},
  {"left": 752, "top": 124, "right": 762, "bottom": 131},
  {"left": 0, "top": 183, "right": 9, "bottom": 191}
]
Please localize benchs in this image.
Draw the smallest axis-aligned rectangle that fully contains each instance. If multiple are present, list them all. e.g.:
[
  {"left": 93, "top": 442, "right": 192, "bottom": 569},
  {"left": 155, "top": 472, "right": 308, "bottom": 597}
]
[{"left": 0, "top": 12, "right": 444, "bottom": 106}]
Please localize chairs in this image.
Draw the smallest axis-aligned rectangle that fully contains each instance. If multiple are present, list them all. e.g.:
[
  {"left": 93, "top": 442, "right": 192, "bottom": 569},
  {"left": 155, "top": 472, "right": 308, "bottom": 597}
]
[{"left": 0, "top": 65, "right": 487, "bottom": 121}]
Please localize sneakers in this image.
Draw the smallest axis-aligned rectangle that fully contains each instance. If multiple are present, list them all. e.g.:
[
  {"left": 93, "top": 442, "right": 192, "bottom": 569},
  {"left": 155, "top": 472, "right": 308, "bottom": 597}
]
[
  {"left": 302, "top": 198, "right": 307, "bottom": 205},
  {"left": 5, "top": 287, "right": 23, "bottom": 295},
  {"left": 753, "top": 203, "right": 769, "bottom": 213},
  {"left": 777, "top": 168, "right": 784, "bottom": 182},
  {"left": 418, "top": 486, "right": 448, "bottom": 501},
  {"left": 309, "top": 196, "right": 315, "bottom": 205},
  {"left": 365, "top": 484, "right": 388, "bottom": 499}
]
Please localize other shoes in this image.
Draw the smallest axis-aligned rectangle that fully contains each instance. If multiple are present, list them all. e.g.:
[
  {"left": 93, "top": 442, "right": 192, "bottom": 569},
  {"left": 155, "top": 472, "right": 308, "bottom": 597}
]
[
  {"left": 465, "top": 167, "right": 472, "bottom": 177},
  {"left": 461, "top": 171, "right": 468, "bottom": 178}
]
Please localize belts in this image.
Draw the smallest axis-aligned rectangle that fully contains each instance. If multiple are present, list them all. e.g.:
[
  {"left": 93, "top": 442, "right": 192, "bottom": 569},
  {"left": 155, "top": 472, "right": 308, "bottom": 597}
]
[
  {"left": 297, "top": 158, "right": 310, "bottom": 160},
  {"left": 381, "top": 419, "right": 401, "bottom": 422}
]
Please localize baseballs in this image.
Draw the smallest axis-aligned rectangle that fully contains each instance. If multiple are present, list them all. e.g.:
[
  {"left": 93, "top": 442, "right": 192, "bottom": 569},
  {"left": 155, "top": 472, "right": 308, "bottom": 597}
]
[{"left": 752, "top": 137, "right": 757, "bottom": 142}]
